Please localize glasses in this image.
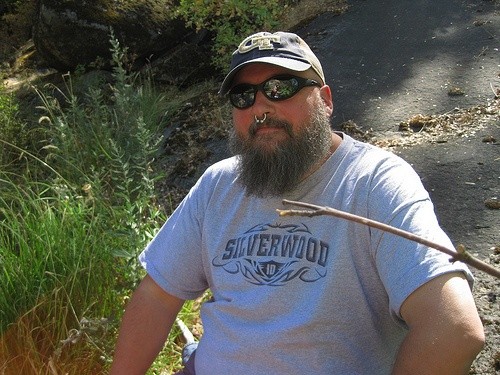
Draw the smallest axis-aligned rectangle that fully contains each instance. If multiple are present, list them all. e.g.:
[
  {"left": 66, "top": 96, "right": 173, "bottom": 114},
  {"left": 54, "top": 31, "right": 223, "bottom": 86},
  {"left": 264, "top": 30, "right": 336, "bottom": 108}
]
[{"left": 227, "top": 73, "right": 323, "bottom": 111}]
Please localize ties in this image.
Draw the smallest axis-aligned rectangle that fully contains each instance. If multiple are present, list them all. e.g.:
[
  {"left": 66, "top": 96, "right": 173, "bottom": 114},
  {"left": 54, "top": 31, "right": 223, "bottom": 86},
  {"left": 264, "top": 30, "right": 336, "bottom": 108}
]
[{"left": 218, "top": 32, "right": 325, "bottom": 93}]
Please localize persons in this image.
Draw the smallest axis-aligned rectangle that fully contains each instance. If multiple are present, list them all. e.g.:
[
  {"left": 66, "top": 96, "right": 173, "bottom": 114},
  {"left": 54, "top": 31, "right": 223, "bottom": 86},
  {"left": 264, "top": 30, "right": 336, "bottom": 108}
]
[{"left": 100, "top": 29, "right": 488, "bottom": 375}]
